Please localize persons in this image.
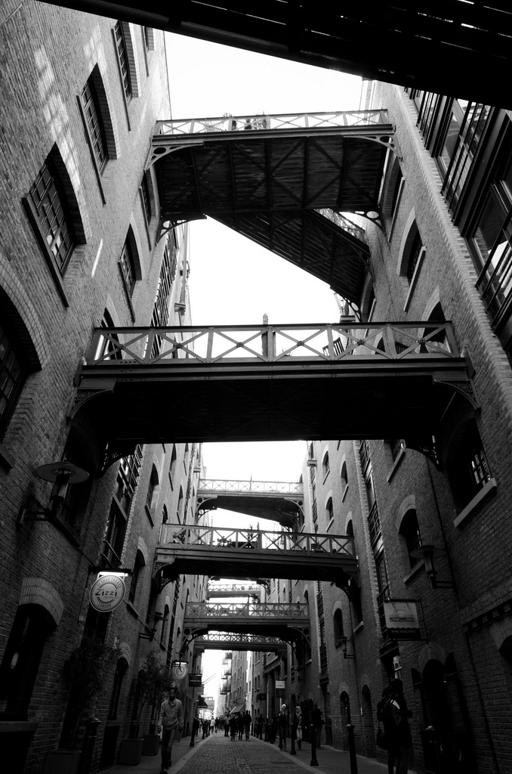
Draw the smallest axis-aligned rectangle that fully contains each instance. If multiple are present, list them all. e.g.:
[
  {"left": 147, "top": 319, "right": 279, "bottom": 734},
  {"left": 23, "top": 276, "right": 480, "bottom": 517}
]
[
  {"left": 381, "top": 678, "right": 410, "bottom": 774},
  {"left": 157, "top": 685, "right": 184, "bottom": 774},
  {"left": 377, "top": 688, "right": 390, "bottom": 732},
  {"left": 195, "top": 699, "right": 324, "bottom": 751}
]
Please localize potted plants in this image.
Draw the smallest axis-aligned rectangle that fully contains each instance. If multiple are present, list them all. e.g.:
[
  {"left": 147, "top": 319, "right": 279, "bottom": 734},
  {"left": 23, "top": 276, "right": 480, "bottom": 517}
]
[{"left": 116, "top": 651, "right": 176, "bottom": 767}]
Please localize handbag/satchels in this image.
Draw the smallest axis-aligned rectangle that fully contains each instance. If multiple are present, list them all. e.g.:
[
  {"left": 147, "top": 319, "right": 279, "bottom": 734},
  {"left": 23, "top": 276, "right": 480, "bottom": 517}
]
[{"left": 376, "top": 716, "right": 389, "bottom": 750}]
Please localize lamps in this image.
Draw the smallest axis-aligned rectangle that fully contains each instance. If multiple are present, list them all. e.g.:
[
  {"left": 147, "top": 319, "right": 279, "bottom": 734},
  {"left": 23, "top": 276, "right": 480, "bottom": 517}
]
[
  {"left": 410, "top": 544, "right": 454, "bottom": 591},
  {"left": 18, "top": 459, "right": 90, "bottom": 529}
]
